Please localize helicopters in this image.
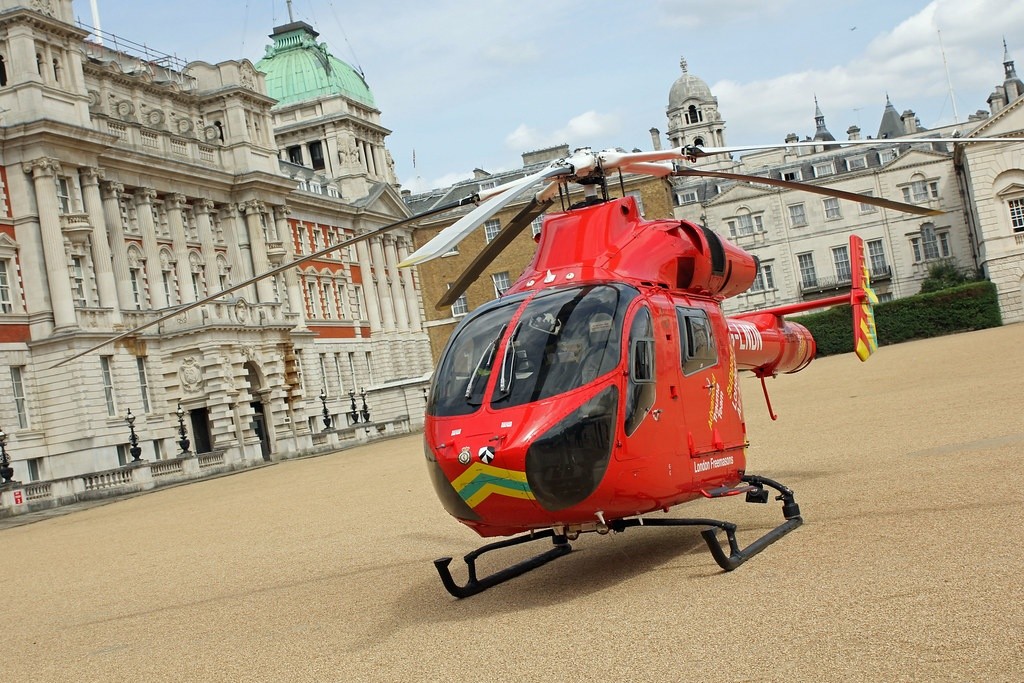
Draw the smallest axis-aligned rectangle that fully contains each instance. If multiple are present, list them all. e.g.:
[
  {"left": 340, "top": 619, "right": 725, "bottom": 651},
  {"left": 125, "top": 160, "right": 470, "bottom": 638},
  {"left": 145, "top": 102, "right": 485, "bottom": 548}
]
[{"left": 45, "top": 131, "right": 1024, "bottom": 598}]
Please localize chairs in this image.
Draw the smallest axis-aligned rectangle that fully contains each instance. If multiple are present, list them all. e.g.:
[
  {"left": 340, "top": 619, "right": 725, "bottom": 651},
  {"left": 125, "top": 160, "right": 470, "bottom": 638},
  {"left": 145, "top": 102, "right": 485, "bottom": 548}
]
[
  {"left": 449, "top": 350, "right": 516, "bottom": 413},
  {"left": 575, "top": 342, "right": 619, "bottom": 419},
  {"left": 588, "top": 313, "right": 615, "bottom": 346}
]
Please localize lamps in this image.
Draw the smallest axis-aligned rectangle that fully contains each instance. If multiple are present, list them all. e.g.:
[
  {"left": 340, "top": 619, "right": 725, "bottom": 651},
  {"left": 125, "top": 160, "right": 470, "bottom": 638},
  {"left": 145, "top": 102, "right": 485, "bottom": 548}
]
[
  {"left": 0, "top": 426, "right": 11, "bottom": 468},
  {"left": 348, "top": 388, "right": 358, "bottom": 414},
  {"left": 124, "top": 408, "right": 139, "bottom": 448},
  {"left": 320, "top": 389, "right": 330, "bottom": 418},
  {"left": 359, "top": 387, "right": 369, "bottom": 413},
  {"left": 175, "top": 403, "right": 188, "bottom": 440}
]
